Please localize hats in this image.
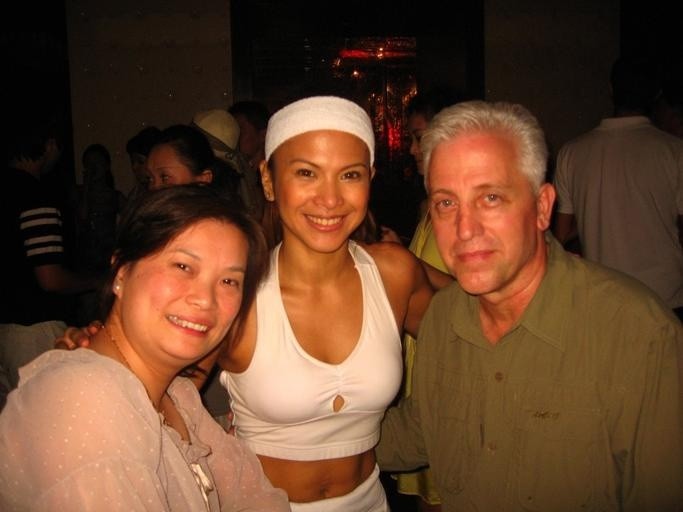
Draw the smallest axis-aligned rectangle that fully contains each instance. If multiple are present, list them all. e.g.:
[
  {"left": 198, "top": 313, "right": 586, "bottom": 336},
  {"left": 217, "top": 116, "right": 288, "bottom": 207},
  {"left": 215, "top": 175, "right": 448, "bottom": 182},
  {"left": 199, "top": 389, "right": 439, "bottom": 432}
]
[{"left": 189, "top": 109, "right": 244, "bottom": 175}]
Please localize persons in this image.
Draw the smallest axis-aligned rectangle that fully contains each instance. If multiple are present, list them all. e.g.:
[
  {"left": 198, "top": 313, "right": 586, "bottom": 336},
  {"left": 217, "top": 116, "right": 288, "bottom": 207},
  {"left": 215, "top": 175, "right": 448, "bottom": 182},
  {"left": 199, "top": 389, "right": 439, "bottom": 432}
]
[
  {"left": 547, "top": 51, "right": 682, "bottom": 334},
  {"left": 51, "top": 72, "right": 439, "bottom": 512},
  {"left": 380, "top": 69, "right": 486, "bottom": 512},
  {"left": 0, "top": 177, "right": 295, "bottom": 512},
  {"left": 1, "top": 93, "right": 425, "bottom": 412},
  {"left": 214, "top": 96, "right": 683, "bottom": 512}
]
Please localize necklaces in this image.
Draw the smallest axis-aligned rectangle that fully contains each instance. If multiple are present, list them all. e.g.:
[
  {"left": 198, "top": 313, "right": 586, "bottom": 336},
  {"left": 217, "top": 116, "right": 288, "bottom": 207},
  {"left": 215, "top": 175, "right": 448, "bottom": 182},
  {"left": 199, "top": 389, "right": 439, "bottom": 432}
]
[{"left": 102, "top": 325, "right": 170, "bottom": 429}]
